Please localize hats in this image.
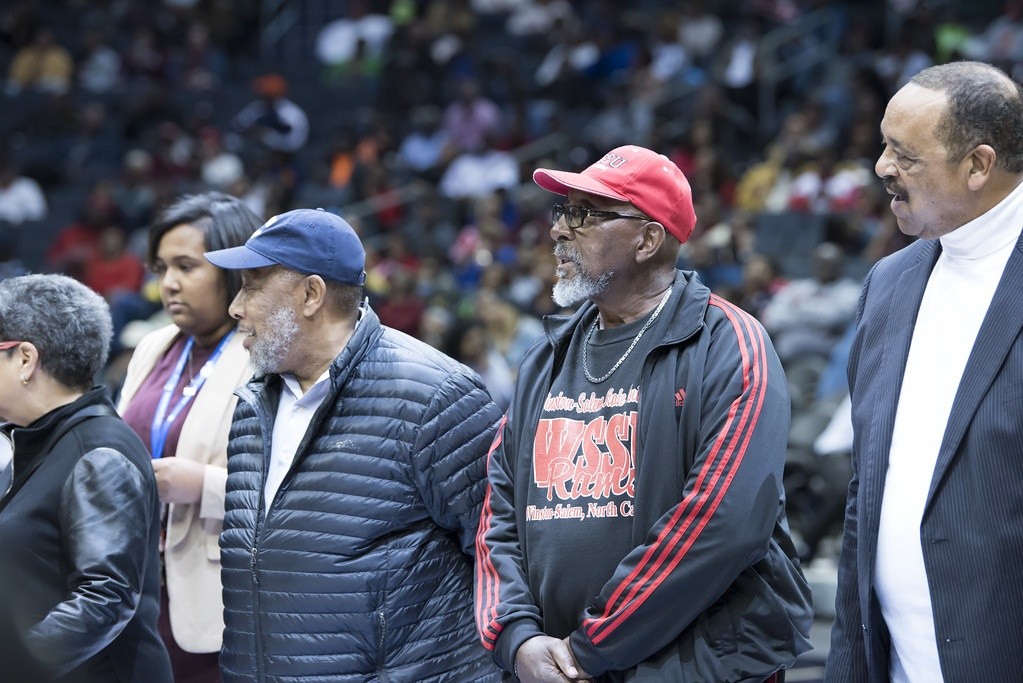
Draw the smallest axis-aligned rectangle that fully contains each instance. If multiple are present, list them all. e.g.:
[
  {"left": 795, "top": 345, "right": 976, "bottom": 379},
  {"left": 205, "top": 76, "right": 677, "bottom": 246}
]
[
  {"left": 203, "top": 208, "right": 367, "bottom": 286},
  {"left": 532, "top": 144, "right": 697, "bottom": 244}
]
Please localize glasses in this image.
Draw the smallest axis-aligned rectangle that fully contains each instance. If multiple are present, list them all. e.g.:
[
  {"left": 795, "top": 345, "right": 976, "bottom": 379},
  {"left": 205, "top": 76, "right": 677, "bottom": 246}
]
[{"left": 551, "top": 204, "right": 652, "bottom": 228}]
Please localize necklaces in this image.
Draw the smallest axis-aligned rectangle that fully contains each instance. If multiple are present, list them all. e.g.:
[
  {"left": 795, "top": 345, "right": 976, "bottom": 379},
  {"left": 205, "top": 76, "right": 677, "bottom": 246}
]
[
  {"left": 583, "top": 284, "right": 673, "bottom": 383},
  {"left": 184, "top": 349, "right": 223, "bottom": 396}
]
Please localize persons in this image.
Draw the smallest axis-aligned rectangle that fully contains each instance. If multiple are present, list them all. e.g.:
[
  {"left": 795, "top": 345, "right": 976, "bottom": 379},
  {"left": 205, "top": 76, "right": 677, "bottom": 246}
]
[
  {"left": 0, "top": 274, "right": 174, "bottom": 683},
  {"left": 203, "top": 208, "right": 505, "bottom": 683},
  {"left": 0, "top": 0, "right": 1023, "bottom": 567},
  {"left": 115, "top": 193, "right": 265, "bottom": 683},
  {"left": 473, "top": 145, "right": 815, "bottom": 683},
  {"left": 825, "top": 62, "right": 1023, "bottom": 682}
]
